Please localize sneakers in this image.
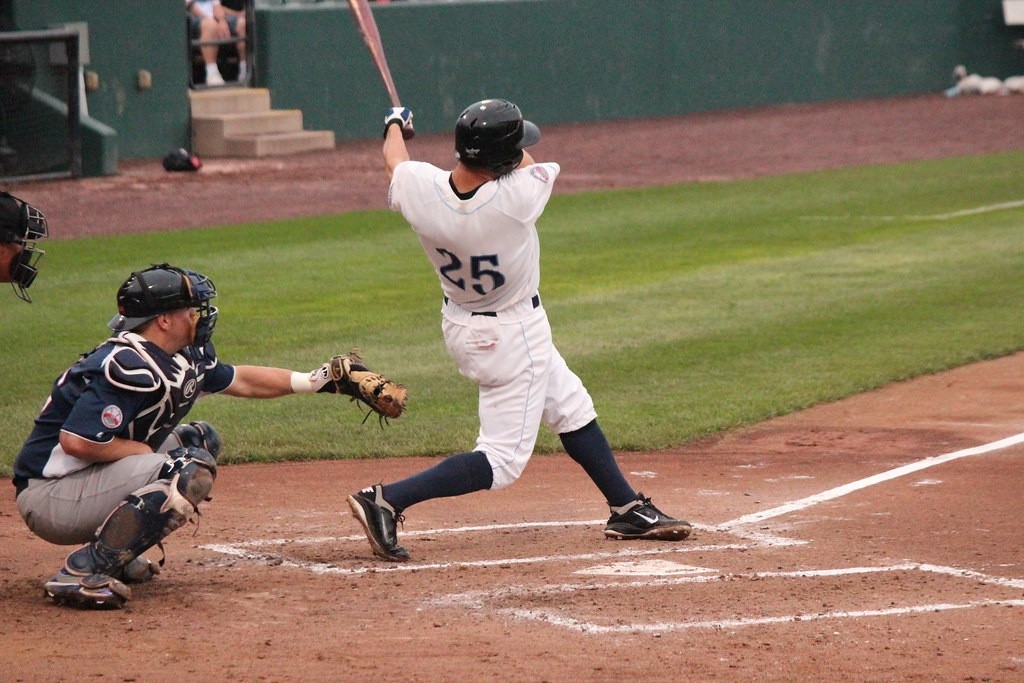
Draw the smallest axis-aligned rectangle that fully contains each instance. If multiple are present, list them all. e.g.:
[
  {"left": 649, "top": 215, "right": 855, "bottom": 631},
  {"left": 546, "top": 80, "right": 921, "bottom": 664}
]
[
  {"left": 603, "top": 491, "right": 692, "bottom": 542},
  {"left": 347, "top": 484, "right": 410, "bottom": 563},
  {"left": 43, "top": 565, "right": 123, "bottom": 608}
]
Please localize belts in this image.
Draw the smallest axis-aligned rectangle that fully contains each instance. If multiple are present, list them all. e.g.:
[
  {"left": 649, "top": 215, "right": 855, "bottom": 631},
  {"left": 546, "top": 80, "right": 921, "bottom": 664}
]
[
  {"left": 14, "top": 477, "right": 45, "bottom": 499},
  {"left": 440, "top": 295, "right": 545, "bottom": 325}
]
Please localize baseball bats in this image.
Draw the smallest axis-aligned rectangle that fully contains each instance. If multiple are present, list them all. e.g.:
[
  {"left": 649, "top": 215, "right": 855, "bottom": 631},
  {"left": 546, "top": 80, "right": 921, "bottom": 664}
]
[{"left": 349, "top": 0, "right": 415, "bottom": 140}]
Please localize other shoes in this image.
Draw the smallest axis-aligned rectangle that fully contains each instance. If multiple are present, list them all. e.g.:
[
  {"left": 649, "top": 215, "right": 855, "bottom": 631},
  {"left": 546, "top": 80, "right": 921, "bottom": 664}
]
[
  {"left": 206, "top": 75, "right": 224, "bottom": 85},
  {"left": 239, "top": 74, "right": 248, "bottom": 85}
]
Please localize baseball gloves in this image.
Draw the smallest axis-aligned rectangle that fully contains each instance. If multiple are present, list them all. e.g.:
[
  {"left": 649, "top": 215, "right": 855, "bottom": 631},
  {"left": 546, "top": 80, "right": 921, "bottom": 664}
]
[{"left": 314, "top": 348, "right": 409, "bottom": 429}]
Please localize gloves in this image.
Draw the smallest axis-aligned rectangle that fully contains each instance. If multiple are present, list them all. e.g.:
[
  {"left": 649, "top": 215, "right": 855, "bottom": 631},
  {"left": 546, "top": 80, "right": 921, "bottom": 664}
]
[{"left": 383, "top": 106, "right": 414, "bottom": 139}]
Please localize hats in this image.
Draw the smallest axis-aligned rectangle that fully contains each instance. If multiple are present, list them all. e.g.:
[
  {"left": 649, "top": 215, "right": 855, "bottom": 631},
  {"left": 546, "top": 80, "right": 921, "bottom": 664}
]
[{"left": 0, "top": 193, "right": 41, "bottom": 244}]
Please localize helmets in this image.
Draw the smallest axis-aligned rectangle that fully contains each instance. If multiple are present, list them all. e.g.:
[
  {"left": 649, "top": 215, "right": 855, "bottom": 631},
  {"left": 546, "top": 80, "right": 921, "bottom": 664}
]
[
  {"left": 455, "top": 98, "right": 540, "bottom": 178},
  {"left": 106, "top": 261, "right": 200, "bottom": 331}
]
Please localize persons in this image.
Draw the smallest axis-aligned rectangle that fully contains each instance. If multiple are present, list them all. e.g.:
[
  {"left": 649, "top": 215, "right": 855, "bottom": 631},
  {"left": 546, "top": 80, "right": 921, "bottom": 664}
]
[
  {"left": 0, "top": 186, "right": 49, "bottom": 292},
  {"left": 347, "top": 99, "right": 693, "bottom": 563},
  {"left": 11, "top": 260, "right": 357, "bottom": 605},
  {"left": 186, "top": 0, "right": 252, "bottom": 86}
]
